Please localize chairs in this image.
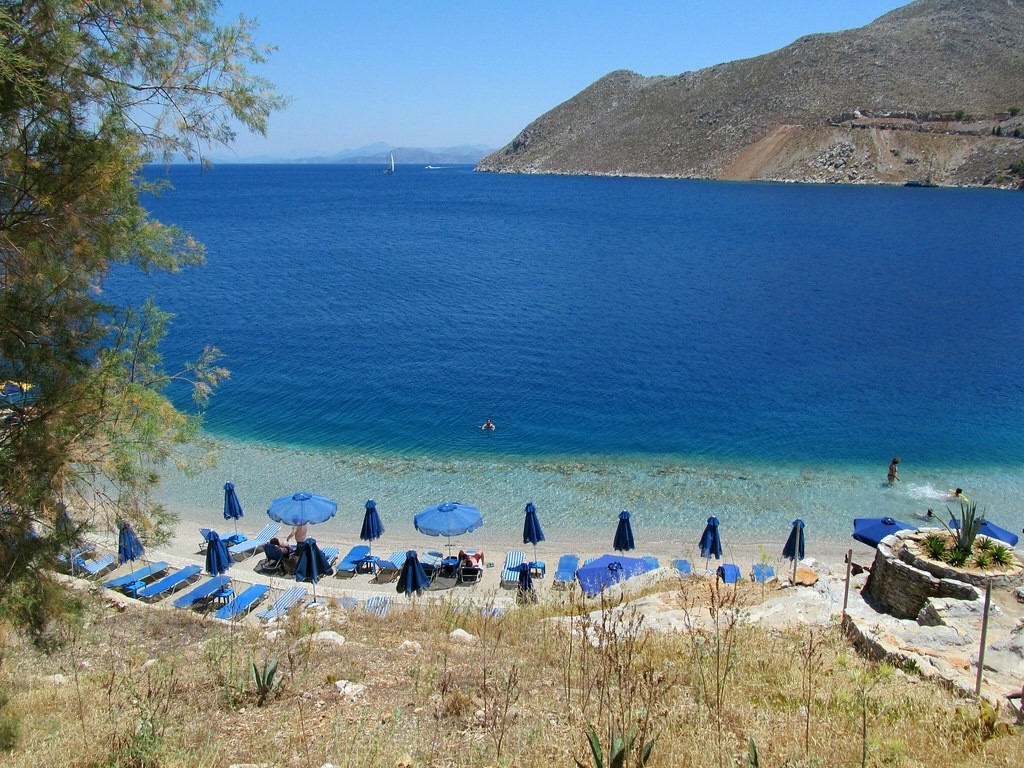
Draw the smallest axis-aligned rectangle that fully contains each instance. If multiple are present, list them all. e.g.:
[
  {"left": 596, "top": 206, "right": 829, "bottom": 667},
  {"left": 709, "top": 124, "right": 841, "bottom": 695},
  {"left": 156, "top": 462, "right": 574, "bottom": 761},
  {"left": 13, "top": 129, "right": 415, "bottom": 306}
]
[{"left": 53, "top": 523, "right": 775, "bottom": 621}]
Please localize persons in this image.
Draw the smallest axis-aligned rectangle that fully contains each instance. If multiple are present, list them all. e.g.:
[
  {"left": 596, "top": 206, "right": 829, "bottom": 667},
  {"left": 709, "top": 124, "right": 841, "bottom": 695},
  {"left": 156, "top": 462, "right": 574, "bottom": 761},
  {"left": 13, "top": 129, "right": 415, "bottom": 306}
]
[
  {"left": 27, "top": 524, "right": 39, "bottom": 539},
  {"left": 457, "top": 549, "right": 484, "bottom": 569},
  {"left": 270, "top": 524, "right": 307, "bottom": 568},
  {"left": 942, "top": 487, "right": 963, "bottom": 499},
  {"left": 913, "top": 509, "right": 933, "bottom": 521},
  {"left": 483, "top": 420, "right": 495, "bottom": 430},
  {"left": 888, "top": 457, "right": 901, "bottom": 482}
]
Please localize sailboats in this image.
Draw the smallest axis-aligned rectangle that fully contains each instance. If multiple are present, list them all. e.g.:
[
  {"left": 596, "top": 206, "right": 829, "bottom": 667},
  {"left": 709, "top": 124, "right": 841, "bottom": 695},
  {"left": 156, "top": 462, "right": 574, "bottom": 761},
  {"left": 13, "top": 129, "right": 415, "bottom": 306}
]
[{"left": 384, "top": 153, "right": 394, "bottom": 173}]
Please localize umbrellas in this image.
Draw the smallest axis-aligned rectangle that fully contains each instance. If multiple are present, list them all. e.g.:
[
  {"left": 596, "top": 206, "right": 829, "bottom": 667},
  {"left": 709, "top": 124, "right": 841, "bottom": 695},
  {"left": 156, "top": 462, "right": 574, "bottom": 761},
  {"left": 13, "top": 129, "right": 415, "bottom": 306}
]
[
  {"left": 205, "top": 529, "right": 233, "bottom": 593},
  {"left": 515, "top": 563, "right": 538, "bottom": 606},
  {"left": 55, "top": 503, "right": 76, "bottom": 533},
  {"left": 118, "top": 522, "right": 146, "bottom": 574},
  {"left": 360, "top": 498, "right": 384, "bottom": 558},
  {"left": 698, "top": 515, "right": 722, "bottom": 570},
  {"left": 784, "top": 519, "right": 805, "bottom": 569},
  {"left": 267, "top": 491, "right": 338, "bottom": 527},
  {"left": 223, "top": 480, "right": 245, "bottom": 536},
  {"left": 414, "top": 501, "right": 483, "bottom": 559},
  {"left": 949, "top": 516, "right": 1018, "bottom": 546},
  {"left": 295, "top": 537, "right": 333, "bottom": 603},
  {"left": 523, "top": 503, "right": 546, "bottom": 561},
  {"left": 576, "top": 510, "right": 647, "bottom": 596},
  {"left": 852, "top": 516, "right": 918, "bottom": 549},
  {"left": 396, "top": 550, "right": 430, "bottom": 596}
]
